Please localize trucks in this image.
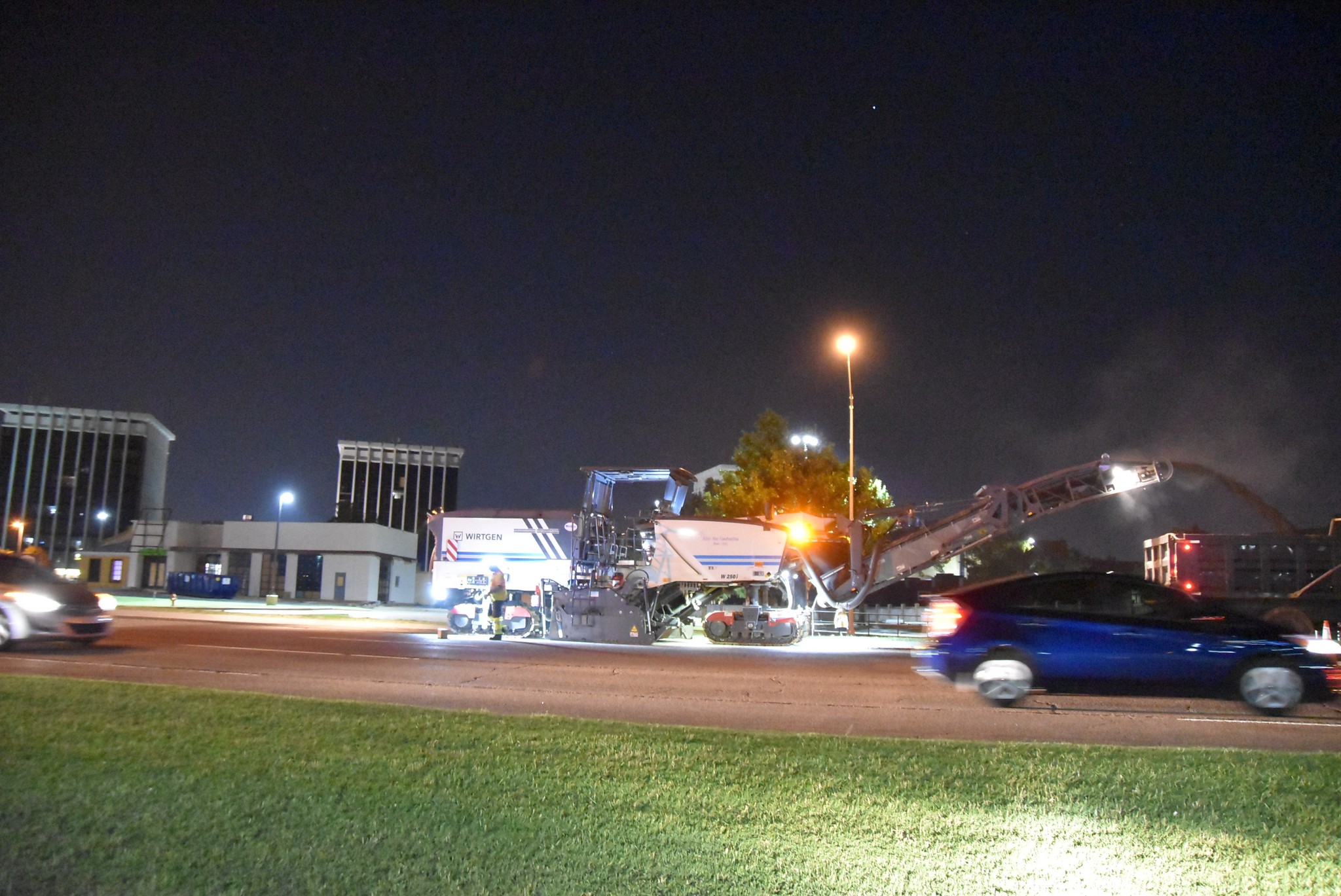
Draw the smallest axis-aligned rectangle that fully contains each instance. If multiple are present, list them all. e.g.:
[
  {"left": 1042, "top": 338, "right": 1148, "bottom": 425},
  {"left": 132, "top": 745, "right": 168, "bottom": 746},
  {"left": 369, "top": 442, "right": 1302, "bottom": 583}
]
[{"left": 1143, "top": 518, "right": 1341, "bottom": 646}]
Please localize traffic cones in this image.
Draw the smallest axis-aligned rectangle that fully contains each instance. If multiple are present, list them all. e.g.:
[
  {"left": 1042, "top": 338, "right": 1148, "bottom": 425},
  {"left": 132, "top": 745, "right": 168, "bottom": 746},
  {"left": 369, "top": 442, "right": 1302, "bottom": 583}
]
[{"left": 1322, "top": 620, "right": 1332, "bottom": 640}]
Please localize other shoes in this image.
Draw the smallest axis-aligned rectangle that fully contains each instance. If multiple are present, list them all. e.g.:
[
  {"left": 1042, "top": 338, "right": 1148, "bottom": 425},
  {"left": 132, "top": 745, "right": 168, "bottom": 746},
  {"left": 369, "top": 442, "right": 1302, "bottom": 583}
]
[{"left": 489, "top": 634, "right": 502, "bottom": 640}]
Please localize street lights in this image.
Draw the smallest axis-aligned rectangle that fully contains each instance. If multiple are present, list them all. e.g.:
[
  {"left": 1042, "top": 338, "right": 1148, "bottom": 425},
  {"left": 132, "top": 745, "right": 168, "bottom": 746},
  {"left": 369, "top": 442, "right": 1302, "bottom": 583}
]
[
  {"left": 836, "top": 335, "right": 856, "bottom": 520},
  {"left": 269, "top": 493, "right": 295, "bottom": 595}
]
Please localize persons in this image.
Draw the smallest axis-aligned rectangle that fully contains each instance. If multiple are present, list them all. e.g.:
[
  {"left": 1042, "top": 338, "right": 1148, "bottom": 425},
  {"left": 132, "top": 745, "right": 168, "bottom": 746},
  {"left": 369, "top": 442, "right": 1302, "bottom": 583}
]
[{"left": 484, "top": 555, "right": 507, "bottom": 640}]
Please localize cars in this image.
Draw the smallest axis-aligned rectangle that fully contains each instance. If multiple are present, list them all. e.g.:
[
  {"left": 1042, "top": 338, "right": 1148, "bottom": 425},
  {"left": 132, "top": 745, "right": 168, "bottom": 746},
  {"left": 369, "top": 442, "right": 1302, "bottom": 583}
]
[
  {"left": 918, "top": 571, "right": 1341, "bottom": 715},
  {"left": 0, "top": 552, "right": 114, "bottom": 652}
]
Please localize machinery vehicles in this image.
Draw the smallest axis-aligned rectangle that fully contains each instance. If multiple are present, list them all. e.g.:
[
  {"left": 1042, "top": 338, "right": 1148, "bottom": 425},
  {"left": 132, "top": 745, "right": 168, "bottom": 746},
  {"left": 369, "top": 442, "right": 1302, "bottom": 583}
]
[{"left": 428, "top": 461, "right": 1174, "bottom": 645}]
